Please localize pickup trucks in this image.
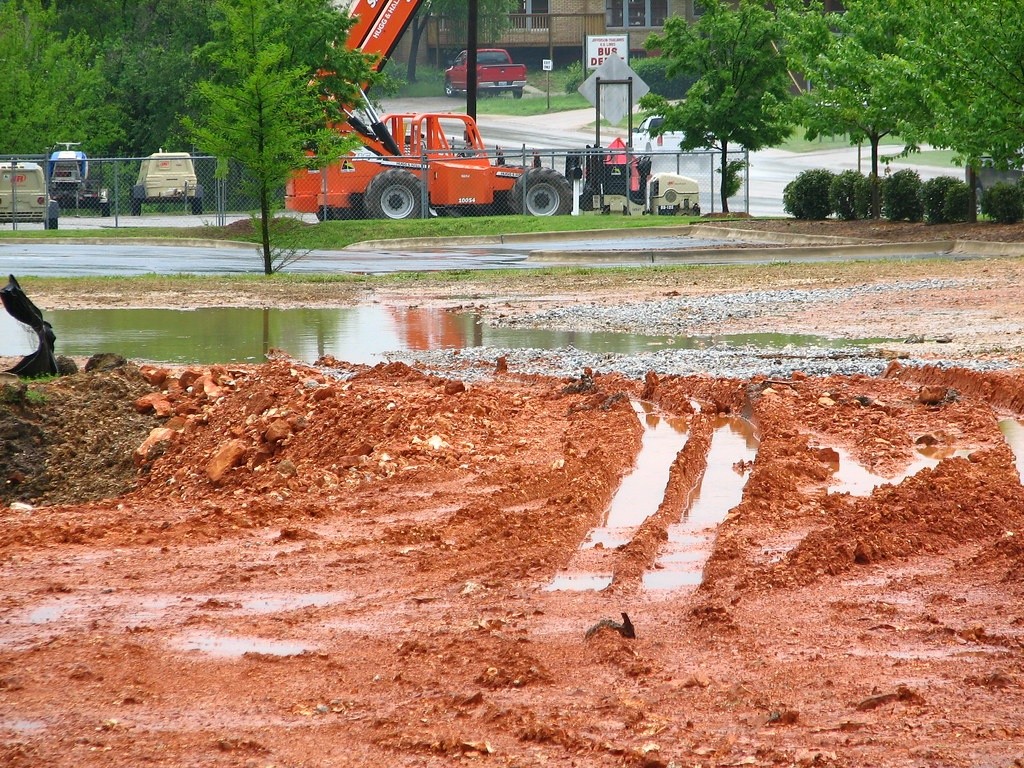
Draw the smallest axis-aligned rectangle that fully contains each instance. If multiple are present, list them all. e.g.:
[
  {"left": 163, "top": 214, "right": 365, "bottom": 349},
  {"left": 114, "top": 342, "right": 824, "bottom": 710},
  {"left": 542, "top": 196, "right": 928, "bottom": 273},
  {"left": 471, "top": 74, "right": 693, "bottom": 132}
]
[
  {"left": 632, "top": 114, "right": 696, "bottom": 156},
  {"left": 442, "top": 49, "right": 526, "bottom": 101},
  {"left": 966, "top": 154, "right": 1024, "bottom": 216}
]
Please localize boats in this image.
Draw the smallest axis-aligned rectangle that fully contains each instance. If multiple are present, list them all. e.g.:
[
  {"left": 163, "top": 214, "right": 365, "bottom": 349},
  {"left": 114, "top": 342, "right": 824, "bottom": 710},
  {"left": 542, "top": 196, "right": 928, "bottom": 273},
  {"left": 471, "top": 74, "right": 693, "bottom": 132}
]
[{"left": 46, "top": 142, "right": 89, "bottom": 188}]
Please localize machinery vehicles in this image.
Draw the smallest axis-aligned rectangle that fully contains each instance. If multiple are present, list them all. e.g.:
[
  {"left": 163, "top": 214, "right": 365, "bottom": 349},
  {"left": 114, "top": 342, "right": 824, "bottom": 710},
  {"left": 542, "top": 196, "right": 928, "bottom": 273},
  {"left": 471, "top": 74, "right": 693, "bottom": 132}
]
[
  {"left": 565, "top": 77, "right": 701, "bottom": 218},
  {"left": 285, "top": 1, "right": 572, "bottom": 219}
]
